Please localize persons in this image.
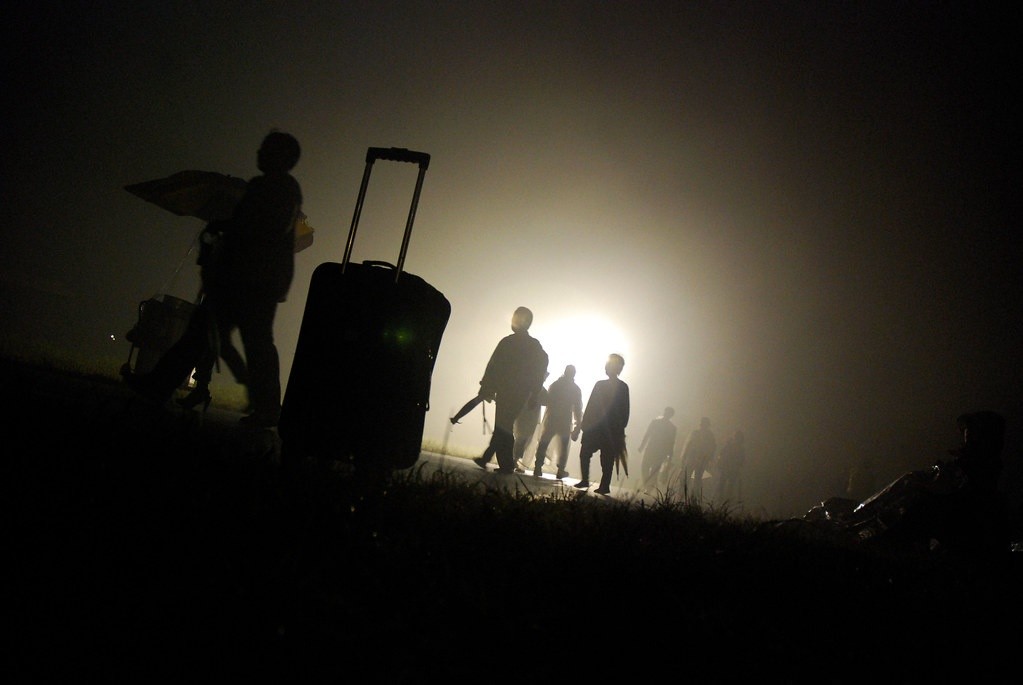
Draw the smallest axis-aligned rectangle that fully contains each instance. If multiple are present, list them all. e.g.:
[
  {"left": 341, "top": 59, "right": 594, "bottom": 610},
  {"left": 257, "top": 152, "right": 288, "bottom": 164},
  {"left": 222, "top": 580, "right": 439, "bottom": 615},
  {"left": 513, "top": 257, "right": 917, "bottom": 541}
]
[
  {"left": 681, "top": 417, "right": 745, "bottom": 505},
  {"left": 637, "top": 406, "right": 677, "bottom": 495},
  {"left": 173, "top": 128, "right": 303, "bottom": 428},
  {"left": 472, "top": 307, "right": 630, "bottom": 494}
]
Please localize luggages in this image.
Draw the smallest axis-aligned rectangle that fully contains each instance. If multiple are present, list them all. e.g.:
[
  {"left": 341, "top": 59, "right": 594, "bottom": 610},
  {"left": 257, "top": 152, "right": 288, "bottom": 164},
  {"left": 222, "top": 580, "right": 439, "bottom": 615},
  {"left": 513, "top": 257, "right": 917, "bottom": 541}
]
[{"left": 279, "top": 147, "right": 452, "bottom": 479}]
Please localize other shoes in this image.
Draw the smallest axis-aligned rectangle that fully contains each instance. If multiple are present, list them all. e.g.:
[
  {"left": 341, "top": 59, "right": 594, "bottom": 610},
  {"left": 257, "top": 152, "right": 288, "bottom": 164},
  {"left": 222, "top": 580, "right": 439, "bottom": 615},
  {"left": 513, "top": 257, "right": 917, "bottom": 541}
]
[
  {"left": 473, "top": 457, "right": 486, "bottom": 469},
  {"left": 534, "top": 473, "right": 542, "bottom": 476},
  {"left": 494, "top": 469, "right": 511, "bottom": 475},
  {"left": 513, "top": 464, "right": 525, "bottom": 474},
  {"left": 556, "top": 471, "right": 568, "bottom": 479},
  {"left": 594, "top": 488, "right": 610, "bottom": 494},
  {"left": 574, "top": 481, "right": 589, "bottom": 488}
]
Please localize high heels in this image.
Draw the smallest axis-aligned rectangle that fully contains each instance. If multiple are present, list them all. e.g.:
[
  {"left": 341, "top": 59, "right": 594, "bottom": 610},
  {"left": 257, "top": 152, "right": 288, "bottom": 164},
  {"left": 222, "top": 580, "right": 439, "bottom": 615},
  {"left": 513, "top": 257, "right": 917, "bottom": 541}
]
[
  {"left": 238, "top": 405, "right": 282, "bottom": 430},
  {"left": 179, "top": 390, "right": 212, "bottom": 412}
]
